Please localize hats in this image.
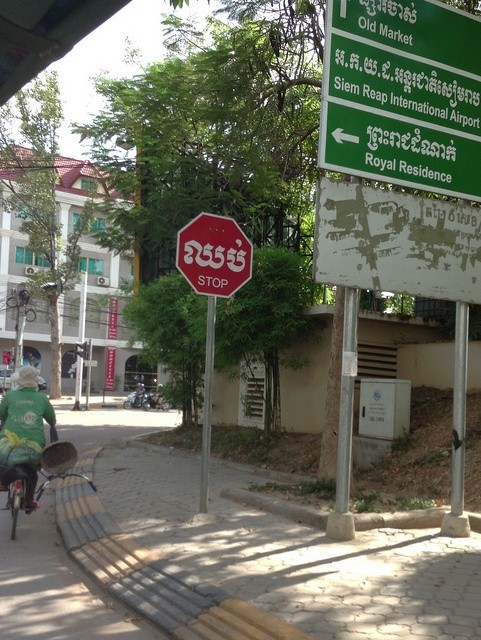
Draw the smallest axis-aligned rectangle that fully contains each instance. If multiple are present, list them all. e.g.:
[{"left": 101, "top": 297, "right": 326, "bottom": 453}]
[{"left": 11, "top": 365, "right": 46, "bottom": 387}]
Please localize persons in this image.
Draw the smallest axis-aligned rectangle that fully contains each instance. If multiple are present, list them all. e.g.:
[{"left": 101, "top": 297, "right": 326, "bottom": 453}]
[
  {"left": 135, "top": 383, "right": 145, "bottom": 407},
  {"left": 152, "top": 384, "right": 165, "bottom": 408},
  {"left": 1, "top": 366, "right": 57, "bottom": 514}
]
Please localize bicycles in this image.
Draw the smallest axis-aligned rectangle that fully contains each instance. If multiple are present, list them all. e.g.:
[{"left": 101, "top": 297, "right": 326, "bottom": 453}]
[
  {"left": 0, "top": 472, "right": 32, "bottom": 540},
  {"left": 34, "top": 425, "right": 97, "bottom": 502}
]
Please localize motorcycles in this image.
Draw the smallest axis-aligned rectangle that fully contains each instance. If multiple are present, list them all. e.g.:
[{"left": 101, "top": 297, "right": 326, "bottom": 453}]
[
  {"left": 123, "top": 390, "right": 142, "bottom": 410},
  {"left": 142, "top": 391, "right": 169, "bottom": 410}
]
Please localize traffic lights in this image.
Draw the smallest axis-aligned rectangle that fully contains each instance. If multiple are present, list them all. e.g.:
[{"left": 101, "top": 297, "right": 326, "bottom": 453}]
[
  {"left": 77, "top": 338, "right": 98, "bottom": 412},
  {"left": 3, "top": 350, "right": 11, "bottom": 396}
]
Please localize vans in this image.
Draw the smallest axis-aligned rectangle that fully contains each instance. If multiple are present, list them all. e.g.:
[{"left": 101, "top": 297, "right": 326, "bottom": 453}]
[{"left": 0, "top": 369, "right": 47, "bottom": 392}]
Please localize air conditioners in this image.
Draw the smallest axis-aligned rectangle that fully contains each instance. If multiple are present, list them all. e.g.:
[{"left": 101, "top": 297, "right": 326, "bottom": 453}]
[
  {"left": 97, "top": 276, "right": 110, "bottom": 287},
  {"left": 25, "top": 266, "right": 39, "bottom": 275}
]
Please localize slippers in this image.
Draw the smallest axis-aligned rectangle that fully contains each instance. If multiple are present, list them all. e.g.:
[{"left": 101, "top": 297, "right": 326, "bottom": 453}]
[{"left": 24, "top": 501, "right": 41, "bottom": 515}]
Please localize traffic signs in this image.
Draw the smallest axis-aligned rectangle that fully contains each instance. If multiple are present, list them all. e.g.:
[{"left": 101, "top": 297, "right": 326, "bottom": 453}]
[
  {"left": 316, "top": 0, "right": 481, "bottom": 305},
  {"left": 178, "top": 213, "right": 255, "bottom": 299}
]
